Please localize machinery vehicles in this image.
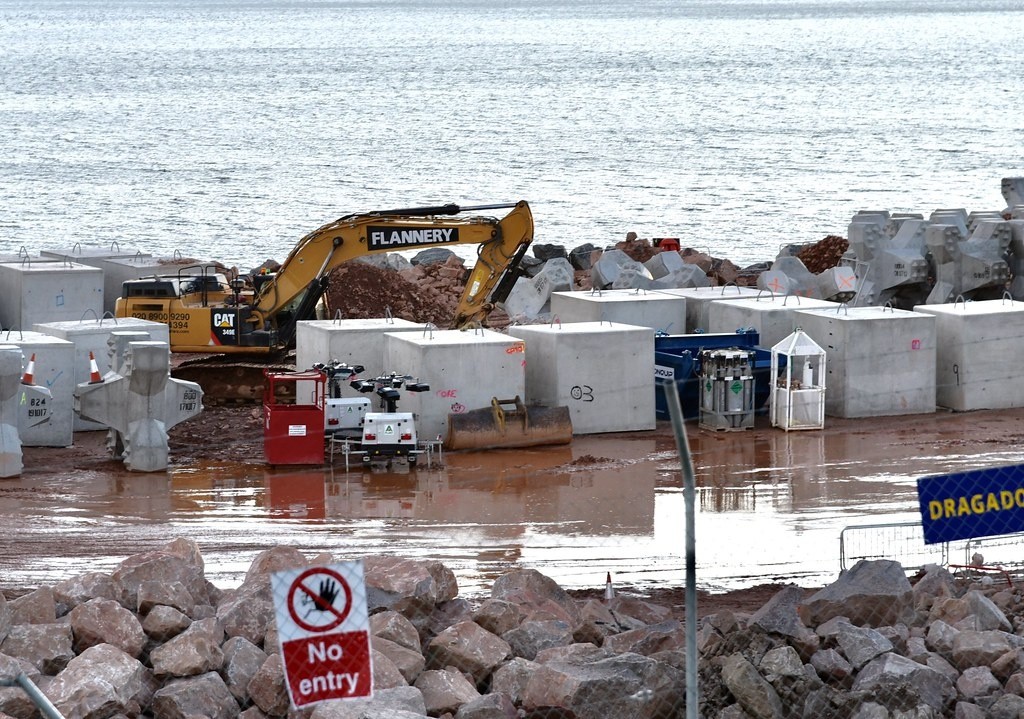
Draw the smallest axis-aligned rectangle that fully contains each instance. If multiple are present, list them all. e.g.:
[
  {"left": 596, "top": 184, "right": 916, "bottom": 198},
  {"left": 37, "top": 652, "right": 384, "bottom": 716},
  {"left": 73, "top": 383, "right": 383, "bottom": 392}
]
[{"left": 115, "top": 199, "right": 540, "bottom": 412}]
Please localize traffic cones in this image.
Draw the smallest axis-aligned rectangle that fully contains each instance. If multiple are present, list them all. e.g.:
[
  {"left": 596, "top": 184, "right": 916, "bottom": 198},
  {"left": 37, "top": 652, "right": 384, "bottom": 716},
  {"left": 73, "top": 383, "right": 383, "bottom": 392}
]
[
  {"left": 20, "top": 352, "right": 39, "bottom": 388},
  {"left": 87, "top": 351, "right": 109, "bottom": 386}
]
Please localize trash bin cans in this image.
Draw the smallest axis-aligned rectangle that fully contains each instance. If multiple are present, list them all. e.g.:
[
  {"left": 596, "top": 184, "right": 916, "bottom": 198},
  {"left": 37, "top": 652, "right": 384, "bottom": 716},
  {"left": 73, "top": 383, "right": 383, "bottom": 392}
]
[
  {"left": 769, "top": 325, "right": 827, "bottom": 432},
  {"left": 261, "top": 369, "right": 327, "bottom": 466}
]
